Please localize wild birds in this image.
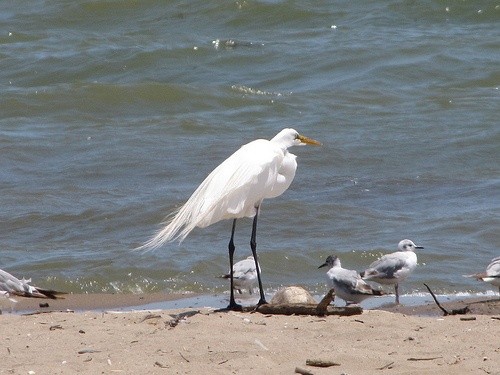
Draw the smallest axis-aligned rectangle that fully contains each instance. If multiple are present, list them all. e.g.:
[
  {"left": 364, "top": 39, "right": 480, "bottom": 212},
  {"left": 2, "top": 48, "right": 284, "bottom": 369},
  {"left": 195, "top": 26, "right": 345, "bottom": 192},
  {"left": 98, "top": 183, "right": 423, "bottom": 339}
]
[
  {"left": 364, "top": 239, "right": 449, "bottom": 316},
  {"left": 479, "top": 257, "right": 500, "bottom": 289},
  {"left": 0, "top": 270, "right": 68, "bottom": 302},
  {"left": 134, "top": 128, "right": 319, "bottom": 311},
  {"left": 221, "top": 256, "right": 262, "bottom": 296},
  {"left": 319, "top": 255, "right": 382, "bottom": 308}
]
